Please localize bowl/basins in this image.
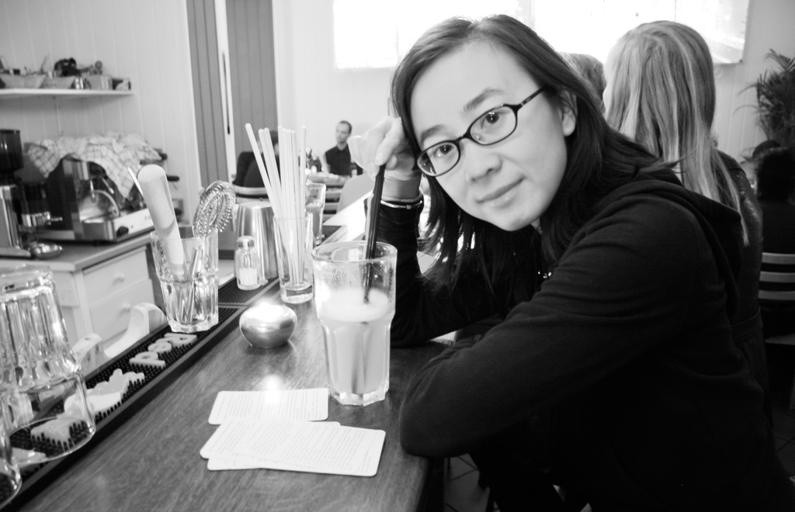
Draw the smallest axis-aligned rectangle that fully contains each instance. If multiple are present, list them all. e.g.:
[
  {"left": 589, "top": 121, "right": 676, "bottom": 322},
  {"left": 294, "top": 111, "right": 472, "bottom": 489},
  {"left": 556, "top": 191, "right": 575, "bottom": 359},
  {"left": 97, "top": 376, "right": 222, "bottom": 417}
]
[{"left": 0, "top": 68, "right": 131, "bottom": 92}]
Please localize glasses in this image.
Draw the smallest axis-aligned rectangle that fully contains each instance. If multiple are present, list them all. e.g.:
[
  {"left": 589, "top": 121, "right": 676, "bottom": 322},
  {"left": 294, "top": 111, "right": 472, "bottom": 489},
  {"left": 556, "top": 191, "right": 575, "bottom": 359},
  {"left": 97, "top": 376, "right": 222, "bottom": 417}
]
[{"left": 417, "top": 87, "right": 544, "bottom": 178}]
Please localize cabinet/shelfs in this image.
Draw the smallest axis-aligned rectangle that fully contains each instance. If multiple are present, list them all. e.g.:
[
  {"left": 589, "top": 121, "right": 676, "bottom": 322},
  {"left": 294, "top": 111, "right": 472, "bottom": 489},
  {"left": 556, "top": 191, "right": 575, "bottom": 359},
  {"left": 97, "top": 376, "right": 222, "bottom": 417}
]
[{"left": 1, "top": 244, "right": 153, "bottom": 354}]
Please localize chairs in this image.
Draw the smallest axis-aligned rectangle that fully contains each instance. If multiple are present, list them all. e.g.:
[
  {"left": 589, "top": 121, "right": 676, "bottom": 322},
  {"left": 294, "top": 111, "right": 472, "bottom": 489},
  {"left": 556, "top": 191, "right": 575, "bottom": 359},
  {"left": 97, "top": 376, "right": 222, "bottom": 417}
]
[
  {"left": 758, "top": 251, "right": 794, "bottom": 349},
  {"left": 226, "top": 180, "right": 268, "bottom": 231}
]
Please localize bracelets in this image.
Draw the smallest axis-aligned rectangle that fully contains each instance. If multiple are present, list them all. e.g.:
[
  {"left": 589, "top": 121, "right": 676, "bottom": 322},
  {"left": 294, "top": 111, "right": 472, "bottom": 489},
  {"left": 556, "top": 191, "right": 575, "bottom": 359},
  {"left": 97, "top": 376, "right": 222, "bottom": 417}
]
[{"left": 380, "top": 200, "right": 422, "bottom": 210}]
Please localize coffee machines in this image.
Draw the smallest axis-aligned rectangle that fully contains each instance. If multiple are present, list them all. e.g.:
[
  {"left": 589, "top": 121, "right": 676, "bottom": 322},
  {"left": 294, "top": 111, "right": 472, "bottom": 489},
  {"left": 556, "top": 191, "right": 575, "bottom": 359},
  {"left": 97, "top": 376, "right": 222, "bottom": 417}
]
[
  {"left": 0, "top": 128, "right": 63, "bottom": 259},
  {"left": 14, "top": 130, "right": 181, "bottom": 245}
]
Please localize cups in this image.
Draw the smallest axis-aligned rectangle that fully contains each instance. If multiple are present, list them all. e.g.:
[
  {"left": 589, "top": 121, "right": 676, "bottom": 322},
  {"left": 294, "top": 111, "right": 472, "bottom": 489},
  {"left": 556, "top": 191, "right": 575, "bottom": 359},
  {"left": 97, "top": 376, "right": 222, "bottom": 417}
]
[
  {"left": 312, "top": 240, "right": 399, "bottom": 408},
  {"left": 148, "top": 223, "right": 220, "bottom": 334},
  {"left": 0, "top": 417, "right": 24, "bottom": 510},
  {"left": 271, "top": 213, "right": 314, "bottom": 305},
  {"left": 306, "top": 182, "right": 327, "bottom": 246},
  {"left": 0, "top": 263, "right": 97, "bottom": 468}
]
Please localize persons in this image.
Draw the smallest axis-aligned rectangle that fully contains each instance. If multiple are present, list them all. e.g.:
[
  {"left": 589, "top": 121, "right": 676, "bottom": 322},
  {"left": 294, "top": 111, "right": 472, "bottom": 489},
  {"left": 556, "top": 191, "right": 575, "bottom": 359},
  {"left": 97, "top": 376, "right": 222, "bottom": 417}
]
[
  {"left": 360, "top": 12, "right": 794, "bottom": 511},
  {"left": 319, "top": 120, "right": 365, "bottom": 178},
  {"left": 600, "top": 23, "right": 774, "bottom": 430},
  {"left": 232, "top": 129, "right": 281, "bottom": 199},
  {"left": 562, "top": 53, "right": 606, "bottom": 100}
]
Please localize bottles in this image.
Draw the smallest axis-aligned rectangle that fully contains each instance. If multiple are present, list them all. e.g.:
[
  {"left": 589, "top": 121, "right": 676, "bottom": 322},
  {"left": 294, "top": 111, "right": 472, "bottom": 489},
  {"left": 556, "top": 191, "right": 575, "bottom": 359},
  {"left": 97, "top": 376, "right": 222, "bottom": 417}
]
[
  {"left": 232, "top": 199, "right": 278, "bottom": 278},
  {"left": 232, "top": 235, "right": 262, "bottom": 291}
]
